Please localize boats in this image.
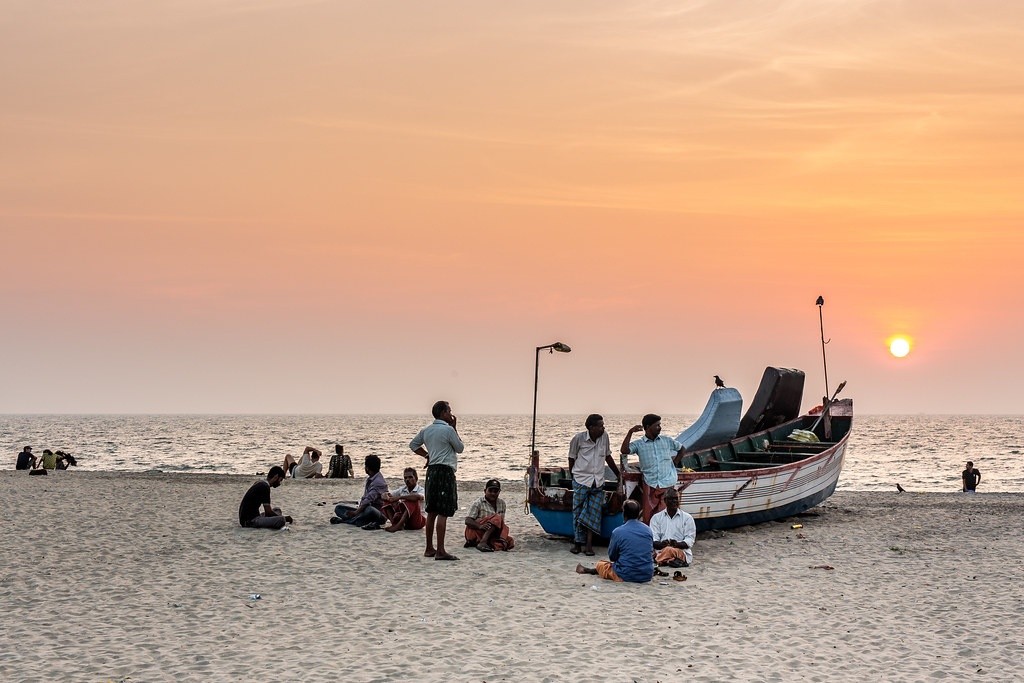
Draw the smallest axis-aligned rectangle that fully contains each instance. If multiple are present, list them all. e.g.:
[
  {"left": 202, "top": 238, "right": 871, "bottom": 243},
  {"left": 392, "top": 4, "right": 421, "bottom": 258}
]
[{"left": 527, "top": 401, "right": 856, "bottom": 547}]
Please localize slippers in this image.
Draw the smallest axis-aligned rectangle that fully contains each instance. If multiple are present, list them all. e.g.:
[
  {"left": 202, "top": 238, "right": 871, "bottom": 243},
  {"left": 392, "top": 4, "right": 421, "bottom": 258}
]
[
  {"left": 476, "top": 544, "right": 494, "bottom": 552},
  {"left": 424, "top": 550, "right": 437, "bottom": 557},
  {"left": 463, "top": 540, "right": 476, "bottom": 548},
  {"left": 435, "top": 554, "right": 461, "bottom": 560}
]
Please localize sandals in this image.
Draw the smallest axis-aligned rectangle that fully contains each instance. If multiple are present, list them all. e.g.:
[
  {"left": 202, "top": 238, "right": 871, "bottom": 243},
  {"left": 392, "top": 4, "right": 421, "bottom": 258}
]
[
  {"left": 673, "top": 571, "right": 687, "bottom": 580},
  {"left": 654, "top": 567, "right": 669, "bottom": 577}
]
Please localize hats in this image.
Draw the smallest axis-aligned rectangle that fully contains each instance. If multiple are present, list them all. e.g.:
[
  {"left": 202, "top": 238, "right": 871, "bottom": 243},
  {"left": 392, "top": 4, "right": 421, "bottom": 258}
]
[{"left": 24, "top": 446, "right": 32, "bottom": 450}]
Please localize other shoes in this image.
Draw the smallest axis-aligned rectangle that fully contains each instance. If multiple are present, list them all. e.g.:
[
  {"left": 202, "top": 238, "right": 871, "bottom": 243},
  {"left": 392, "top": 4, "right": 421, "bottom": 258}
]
[
  {"left": 570, "top": 547, "right": 582, "bottom": 554},
  {"left": 361, "top": 521, "right": 381, "bottom": 530},
  {"left": 356, "top": 517, "right": 371, "bottom": 527},
  {"left": 583, "top": 548, "right": 595, "bottom": 556},
  {"left": 330, "top": 516, "right": 344, "bottom": 524}
]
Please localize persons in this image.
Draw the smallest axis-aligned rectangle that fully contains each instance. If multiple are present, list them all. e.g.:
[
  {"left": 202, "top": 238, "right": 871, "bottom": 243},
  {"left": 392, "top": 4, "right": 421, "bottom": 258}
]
[
  {"left": 382, "top": 467, "right": 426, "bottom": 533},
  {"left": 335, "top": 454, "right": 388, "bottom": 526},
  {"left": 16, "top": 446, "right": 37, "bottom": 470},
  {"left": 568, "top": 414, "right": 619, "bottom": 556},
  {"left": 408, "top": 401, "right": 464, "bottom": 560},
  {"left": 576, "top": 500, "right": 654, "bottom": 582},
  {"left": 650, "top": 488, "right": 696, "bottom": 568},
  {"left": 620, "top": 414, "right": 685, "bottom": 526},
  {"left": 326, "top": 445, "right": 354, "bottom": 478},
  {"left": 283, "top": 446, "right": 323, "bottom": 479},
  {"left": 239, "top": 466, "right": 293, "bottom": 529},
  {"left": 464, "top": 479, "right": 514, "bottom": 553},
  {"left": 962, "top": 461, "right": 981, "bottom": 493},
  {"left": 37, "top": 449, "right": 64, "bottom": 469}
]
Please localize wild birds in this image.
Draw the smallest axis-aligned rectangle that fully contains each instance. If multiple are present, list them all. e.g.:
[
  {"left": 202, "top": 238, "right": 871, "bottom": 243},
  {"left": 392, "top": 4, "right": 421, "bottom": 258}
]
[
  {"left": 895, "top": 483, "right": 906, "bottom": 493},
  {"left": 713, "top": 375, "right": 727, "bottom": 388}
]
